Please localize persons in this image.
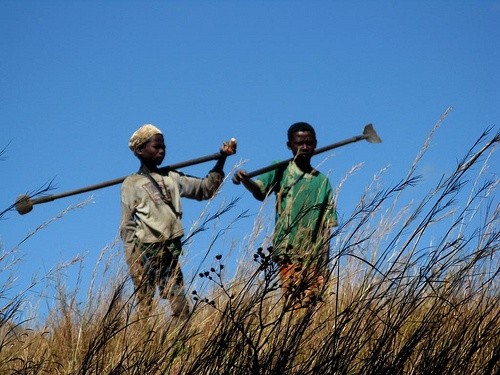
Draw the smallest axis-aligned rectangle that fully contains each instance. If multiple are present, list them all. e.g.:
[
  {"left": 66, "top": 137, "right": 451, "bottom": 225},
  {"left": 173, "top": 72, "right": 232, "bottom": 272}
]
[
  {"left": 231, "top": 121, "right": 339, "bottom": 314},
  {"left": 119, "top": 122, "right": 234, "bottom": 323}
]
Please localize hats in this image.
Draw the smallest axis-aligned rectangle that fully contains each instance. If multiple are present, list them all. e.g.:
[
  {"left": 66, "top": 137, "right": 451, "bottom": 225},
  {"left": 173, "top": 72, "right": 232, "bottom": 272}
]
[{"left": 129, "top": 124, "right": 163, "bottom": 153}]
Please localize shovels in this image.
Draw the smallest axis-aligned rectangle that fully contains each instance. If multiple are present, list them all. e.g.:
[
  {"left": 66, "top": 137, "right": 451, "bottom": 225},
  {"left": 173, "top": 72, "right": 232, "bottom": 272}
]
[
  {"left": 14, "top": 149, "right": 238, "bottom": 216},
  {"left": 239, "top": 122, "right": 382, "bottom": 181}
]
